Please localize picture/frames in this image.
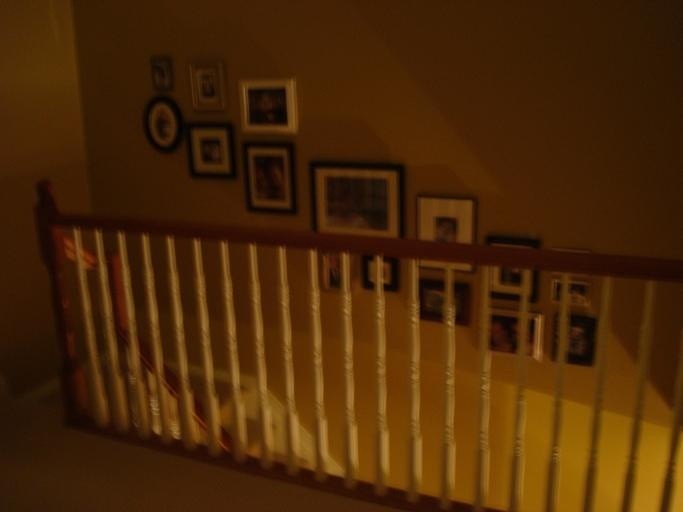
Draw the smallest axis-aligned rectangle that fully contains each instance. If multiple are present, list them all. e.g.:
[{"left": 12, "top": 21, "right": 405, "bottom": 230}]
[
  {"left": 144, "top": 97, "right": 185, "bottom": 151},
  {"left": 238, "top": 75, "right": 302, "bottom": 138},
  {"left": 148, "top": 56, "right": 180, "bottom": 95},
  {"left": 482, "top": 229, "right": 541, "bottom": 306},
  {"left": 548, "top": 310, "right": 599, "bottom": 370},
  {"left": 549, "top": 275, "right": 594, "bottom": 308},
  {"left": 311, "top": 246, "right": 352, "bottom": 295},
  {"left": 185, "top": 121, "right": 237, "bottom": 182},
  {"left": 243, "top": 139, "right": 300, "bottom": 216},
  {"left": 358, "top": 254, "right": 402, "bottom": 293},
  {"left": 417, "top": 275, "right": 473, "bottom": 328},
  {"left": 305, "top": 157, "right": 407, "bottom": 241},
  {"left": 185, "top": 58, "right": 229, "bottom": 113},
  {"left": 415, "top": 187, "right": 477, "bottom": 278},
  {"left": 479, "top": 304, "right": 546, "bottom": 361}
]
